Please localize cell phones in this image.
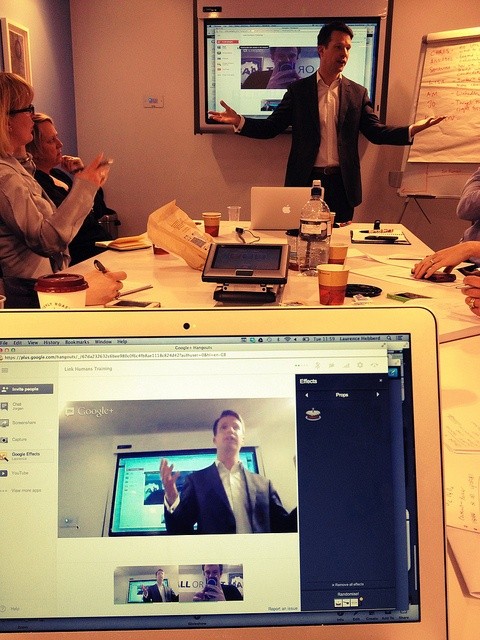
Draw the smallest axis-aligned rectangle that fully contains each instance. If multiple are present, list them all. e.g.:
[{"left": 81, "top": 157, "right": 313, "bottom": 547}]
[
  {"left": 411, "top": 268, "right": 456, "bottom": 281},
  {"left": 279, "top": 62, "right": 295, "bottom": 71},
  {"left": 207, "top": 577, "right": 217, "bottom": 598},
  {"left": 112, "top": 300, "right": 148, "bottom": 308}
]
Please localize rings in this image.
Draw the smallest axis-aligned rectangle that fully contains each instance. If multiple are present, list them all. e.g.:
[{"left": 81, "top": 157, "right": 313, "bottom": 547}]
[
  {"left": 469, "top": 298, "right": 476, "bottom": 309},
  {"left": 429, "top": 260, "right": 434, "bottom": 264}
]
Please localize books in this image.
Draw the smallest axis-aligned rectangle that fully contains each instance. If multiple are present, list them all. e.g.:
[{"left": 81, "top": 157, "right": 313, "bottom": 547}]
[{"left": 94, "top": 235, "right": 153, "bottom": 252}]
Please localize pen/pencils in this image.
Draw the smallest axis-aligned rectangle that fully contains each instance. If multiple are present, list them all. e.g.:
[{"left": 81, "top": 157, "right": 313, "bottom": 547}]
[
  {"left": 94, "top": 260, "right": 107, "bottom": 272},
  {"left": 69, "top": 161, "right": 113, "bottom": 175},
  {"left": 456, "top": 285, "right": 472, "bottom": 289},
  {"left": 359, "top": 228, "right": 394, "bottom": 233}
]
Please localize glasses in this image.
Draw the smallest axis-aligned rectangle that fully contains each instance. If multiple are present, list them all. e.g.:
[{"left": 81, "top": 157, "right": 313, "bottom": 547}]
[{"left": 10, "top": 104, "right": 34, "bottom": 114}]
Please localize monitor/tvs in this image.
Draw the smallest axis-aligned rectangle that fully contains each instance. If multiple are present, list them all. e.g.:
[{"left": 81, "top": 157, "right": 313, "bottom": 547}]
[
  {"left": 128, "top": 579, "right": 168, "bottom": 602},
  {"left": 202, "top": 243, "right": 290, "bottom": 301}
]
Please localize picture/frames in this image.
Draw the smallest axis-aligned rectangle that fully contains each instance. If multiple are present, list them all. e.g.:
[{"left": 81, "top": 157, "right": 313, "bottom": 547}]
[{"left": 1, "top": 16, "right": 34, "bottom": 88}]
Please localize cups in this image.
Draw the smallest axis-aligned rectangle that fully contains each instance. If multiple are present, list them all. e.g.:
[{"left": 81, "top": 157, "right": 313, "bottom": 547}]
[
  {"left": 285, "top": 229, "right": 301, "bottom": 271},
  {"left": 328, "top": 242, "right": 349, "bottom": 265},
  {"left": 228, "top": 205, "right": 241, "bottom": 226},
  {"left": 202, "top": 212, "right": 221, "bottom": 237},
  {"left": 34, "top": 273, "right": 90, "bottom": 310},
  {"left": 316, "top": 263, "right": 350, "bottom": 305}
]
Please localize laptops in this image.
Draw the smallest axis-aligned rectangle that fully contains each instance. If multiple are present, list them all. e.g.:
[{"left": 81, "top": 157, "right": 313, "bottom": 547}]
[
  {"left": 1, "top": 308, "right": 446, "bottom": 640},
  {"left": 251, "top": 187, "right": 325, "bottom": 230}
]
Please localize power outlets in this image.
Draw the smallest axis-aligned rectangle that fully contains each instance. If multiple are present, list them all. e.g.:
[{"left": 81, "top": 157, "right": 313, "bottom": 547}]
[{"left": 141, "top": 93, "right": 164, "bottom": 108}]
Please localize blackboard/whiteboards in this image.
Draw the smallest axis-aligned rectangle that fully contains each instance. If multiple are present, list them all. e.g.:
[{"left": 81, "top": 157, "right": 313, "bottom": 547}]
[{"left": 395, "top": 27, "right": 480, "bottom": 198}]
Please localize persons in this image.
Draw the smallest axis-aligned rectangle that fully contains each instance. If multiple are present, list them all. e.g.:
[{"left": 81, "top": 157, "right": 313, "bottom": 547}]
[
  {"left": 192, "top": 565, "right": 243, "bottom": 600},
  {"left": 411, "top": 240, "right": 480, "bottom": 320},
  {"left": 455, "top": 166, "right": 480, "bottom": 245},
  {"left": 207, "top": 23, "right": 448, "bottom": 222},
  {"left": 159, "top": 409, "right": 296, "bottom": 533},
  {"left": 1, "top": 270, "right": 128, "bottom": 309},
  {"left": 241, "top": 47, "right": 304, "bottom": 89},
  {"left": 141, "top": 569, "right": 179, "bottom": 602},
  {"left": 1, "top": 73, "right": 112, "bottom": 279},
  {"left": 25, "top": 111, "right": 115, "bottom": 270}
]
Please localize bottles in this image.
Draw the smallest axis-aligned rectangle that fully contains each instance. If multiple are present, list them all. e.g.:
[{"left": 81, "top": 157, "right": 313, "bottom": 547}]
[{"left": 297, "top": 186, "right": 332, "bottom": 277}]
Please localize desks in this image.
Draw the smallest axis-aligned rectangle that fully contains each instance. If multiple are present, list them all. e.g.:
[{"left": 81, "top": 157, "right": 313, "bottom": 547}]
[{"left": 20, "top": 222, "right": 480, "bottom": 638}]
